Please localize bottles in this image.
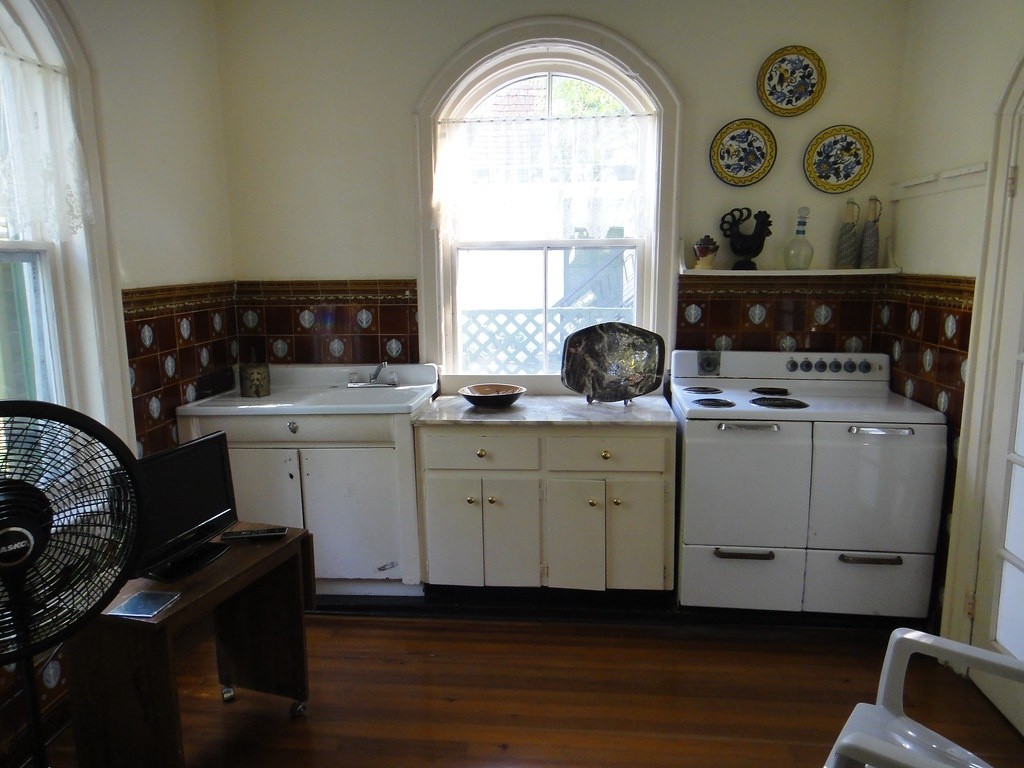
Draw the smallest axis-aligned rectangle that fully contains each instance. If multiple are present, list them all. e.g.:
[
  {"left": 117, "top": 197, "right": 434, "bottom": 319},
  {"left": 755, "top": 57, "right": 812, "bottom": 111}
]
[
  {"left": 858, "top": 196, "right": 883, "bottom": 269},
  {"left": 783, "top": 207, "right": 815, "bottom": 270},
  {"left": 834, "top": 197, "right": 861, "bottom": 269}
]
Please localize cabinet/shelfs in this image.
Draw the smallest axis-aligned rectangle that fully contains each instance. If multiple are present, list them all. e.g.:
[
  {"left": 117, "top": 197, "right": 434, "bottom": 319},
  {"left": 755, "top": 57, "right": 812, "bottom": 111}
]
[
  {"left": 677, "top": 418, "right": 947, "bottom": 618},
  {"left": 63, "top": 520, "right": 317, "bottom": 768},
  {"left": 412, "top": 425, "right": 674, "bottom": 592},
  {"left": 176, "top": 416, "right": 424, "bottom": 598}
]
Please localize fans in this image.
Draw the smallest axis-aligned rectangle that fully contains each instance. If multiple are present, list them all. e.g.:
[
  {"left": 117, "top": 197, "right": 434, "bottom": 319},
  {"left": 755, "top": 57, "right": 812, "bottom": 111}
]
[{"left": 0, "top": 400, "right": 147, "bottom": 768}]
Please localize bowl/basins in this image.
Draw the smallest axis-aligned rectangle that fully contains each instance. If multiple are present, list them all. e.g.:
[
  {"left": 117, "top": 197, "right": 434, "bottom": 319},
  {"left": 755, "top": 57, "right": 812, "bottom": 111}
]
[{"left": 458, "top": 382, "right": 529, "bottom": 409}]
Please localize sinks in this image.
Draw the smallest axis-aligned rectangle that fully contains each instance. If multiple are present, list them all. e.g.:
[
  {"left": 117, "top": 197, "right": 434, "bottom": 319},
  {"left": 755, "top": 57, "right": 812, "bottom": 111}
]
[{"left": 296, "top": 389, "right": 421, "bottom": 404}]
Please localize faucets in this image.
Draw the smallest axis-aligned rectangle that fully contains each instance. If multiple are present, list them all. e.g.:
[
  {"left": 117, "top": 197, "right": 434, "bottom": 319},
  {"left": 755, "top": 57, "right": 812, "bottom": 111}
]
[{"left": 370, "top": 361, "right": 388, "bottom": 384}]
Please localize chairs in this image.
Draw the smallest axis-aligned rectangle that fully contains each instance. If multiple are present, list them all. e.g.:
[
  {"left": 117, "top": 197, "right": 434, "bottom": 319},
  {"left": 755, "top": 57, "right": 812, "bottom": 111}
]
[{"left": 822, "top": 627, "right": 1024, "bottom": 768}]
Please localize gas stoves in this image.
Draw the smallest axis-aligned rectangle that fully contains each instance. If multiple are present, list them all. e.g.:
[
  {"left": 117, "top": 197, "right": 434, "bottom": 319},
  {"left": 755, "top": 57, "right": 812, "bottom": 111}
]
[{"left": 669, "top": 350, "right": 946, "bottom": 424}]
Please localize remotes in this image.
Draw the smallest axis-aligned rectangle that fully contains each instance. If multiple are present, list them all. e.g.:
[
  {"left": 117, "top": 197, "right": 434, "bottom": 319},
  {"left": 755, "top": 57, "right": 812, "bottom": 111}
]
[{"left": 219, "top": 526, "right": 288, "bottom": 543}]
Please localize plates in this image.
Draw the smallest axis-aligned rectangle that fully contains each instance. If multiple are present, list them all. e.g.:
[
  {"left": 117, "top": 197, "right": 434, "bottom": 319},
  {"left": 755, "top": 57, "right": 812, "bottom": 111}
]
[
  {"left": 709, "top": 118, "right": 777, "bottom": 187},
  {"left": 803, "top": 124, "right": 874, "bottom": 194},
  {"left": 756, "top": 45, "right": 827, "bottom": 117}
]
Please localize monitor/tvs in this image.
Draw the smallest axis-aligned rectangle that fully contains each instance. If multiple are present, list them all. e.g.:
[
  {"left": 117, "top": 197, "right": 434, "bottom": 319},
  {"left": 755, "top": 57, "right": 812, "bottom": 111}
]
[{"left": 109, "top": 429, "right": 238, "bottom": 583}]
